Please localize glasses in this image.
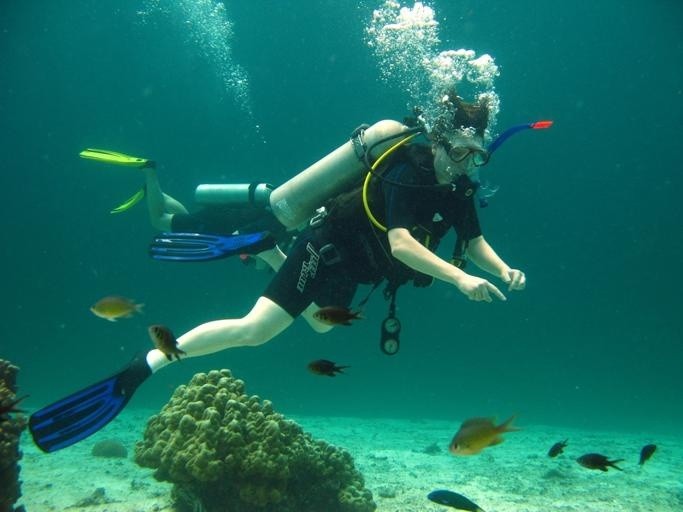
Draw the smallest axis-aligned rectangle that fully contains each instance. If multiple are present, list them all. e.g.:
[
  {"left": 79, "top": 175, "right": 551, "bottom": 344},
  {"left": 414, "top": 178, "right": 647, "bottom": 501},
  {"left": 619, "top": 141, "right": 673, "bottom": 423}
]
[{"left": 439, "top": 138, "right": 489, "bottom": 167}]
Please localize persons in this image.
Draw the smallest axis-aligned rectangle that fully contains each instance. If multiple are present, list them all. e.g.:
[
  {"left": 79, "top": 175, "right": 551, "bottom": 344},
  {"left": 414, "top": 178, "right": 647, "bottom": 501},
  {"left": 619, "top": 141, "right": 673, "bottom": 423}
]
[
  {"left": 28, "top": 88, "right": 553, "bottom": 454},
  {"left": 79, "top": 148, "right": 278, "bottom": 235}
]
